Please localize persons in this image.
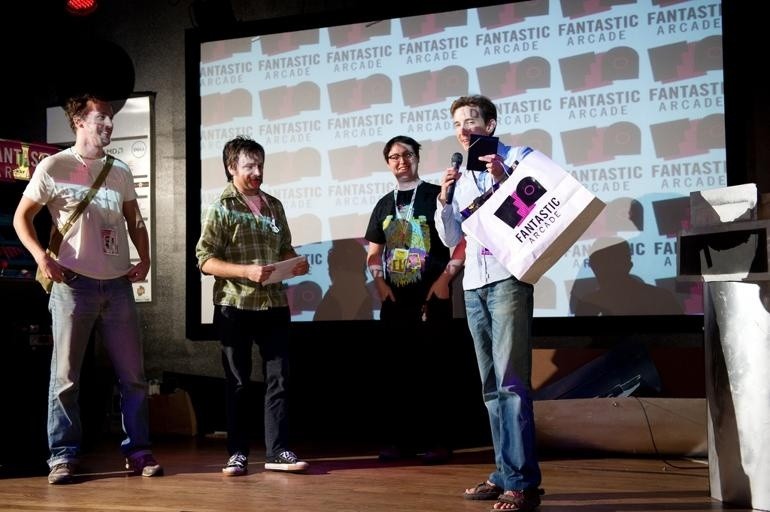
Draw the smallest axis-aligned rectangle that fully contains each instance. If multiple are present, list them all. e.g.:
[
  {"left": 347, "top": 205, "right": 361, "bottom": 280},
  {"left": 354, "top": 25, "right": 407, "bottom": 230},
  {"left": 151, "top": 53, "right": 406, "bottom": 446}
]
[
  {"left": 364, "top": 136, "right": 467, "bottom": 467},
  {"left": 195, "top": 135, "right": 310, "bottom": 477},
  {"left": 12, "top": 91, "right": 162, "bottom": 486},
  {"left": 103, "top": 235, "right": 112, "bottom": 253},
  {"left": 431, "top": 94, "right": 542, "bottom": 511}
]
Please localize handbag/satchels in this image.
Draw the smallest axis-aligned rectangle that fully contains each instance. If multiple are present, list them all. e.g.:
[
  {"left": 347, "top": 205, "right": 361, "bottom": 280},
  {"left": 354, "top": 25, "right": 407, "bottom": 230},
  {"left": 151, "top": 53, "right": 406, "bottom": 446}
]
[
  {"left": 34, "top": 228, "right": 61, "bottom": 294},
  {"left": 461, "top": 147, "right": 606, "bottom": 283}
]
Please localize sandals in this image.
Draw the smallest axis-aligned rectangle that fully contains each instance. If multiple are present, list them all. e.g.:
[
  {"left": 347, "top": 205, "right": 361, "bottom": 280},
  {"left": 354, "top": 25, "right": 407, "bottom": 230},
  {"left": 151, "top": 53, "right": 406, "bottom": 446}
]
[{"left": 463, "top": 481, "right": 544, "bottom": 512}]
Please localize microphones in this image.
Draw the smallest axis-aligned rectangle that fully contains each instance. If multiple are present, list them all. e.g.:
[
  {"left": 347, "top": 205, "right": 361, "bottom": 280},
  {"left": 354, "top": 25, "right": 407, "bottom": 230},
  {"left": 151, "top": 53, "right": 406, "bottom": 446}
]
[{"left": 446, "top": 152, "right": 463, "bottom": 205}]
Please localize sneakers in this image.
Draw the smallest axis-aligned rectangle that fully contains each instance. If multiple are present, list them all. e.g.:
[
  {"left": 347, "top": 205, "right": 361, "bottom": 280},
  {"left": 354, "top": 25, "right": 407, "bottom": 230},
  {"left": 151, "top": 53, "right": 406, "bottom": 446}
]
[
  {"left": 48, "top": 464, "right": 75, "bottom": 483},
  {"left": 125, "top": 454, "right": 164, "bottom": 476},
  {"left": 264, "top": 450, "right": 309, "bottom": 471},
  {"left": 379, "top": 447, "right": 449, "bottom": 465},
  {"left": 222, "top": 452, "right": 248, "bottom": 475}
]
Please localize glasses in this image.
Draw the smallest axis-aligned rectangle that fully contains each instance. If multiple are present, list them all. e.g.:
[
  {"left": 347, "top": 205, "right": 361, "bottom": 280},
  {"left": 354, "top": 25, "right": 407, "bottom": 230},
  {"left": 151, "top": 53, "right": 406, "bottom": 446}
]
[{"left": 388, "top": 152, "right": 417, "bottom": 159}]
